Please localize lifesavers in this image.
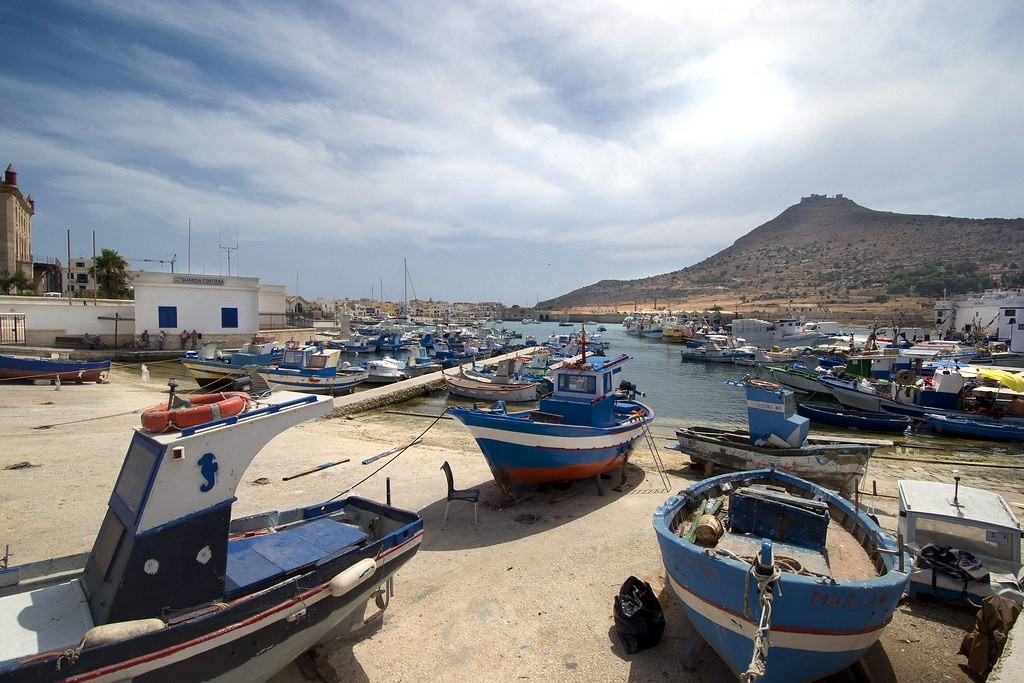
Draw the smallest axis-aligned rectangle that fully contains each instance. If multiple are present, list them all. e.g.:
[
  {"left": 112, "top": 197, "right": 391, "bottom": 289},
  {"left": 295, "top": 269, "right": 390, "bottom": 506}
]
[
  {"left": 288, "top": 346, "right": 300, "bottom": 349},
  {"left": 140, "top": 391, "right": 252, "bottom": 432},
  {"left": 750, "top": 379, "right": 779, "bottom": 389}
]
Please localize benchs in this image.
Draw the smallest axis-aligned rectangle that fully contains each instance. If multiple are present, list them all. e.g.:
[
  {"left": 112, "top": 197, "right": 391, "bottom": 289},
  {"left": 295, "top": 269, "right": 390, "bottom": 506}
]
[{"left": 56, "top": 337, "right": 97, "bottom": 349}]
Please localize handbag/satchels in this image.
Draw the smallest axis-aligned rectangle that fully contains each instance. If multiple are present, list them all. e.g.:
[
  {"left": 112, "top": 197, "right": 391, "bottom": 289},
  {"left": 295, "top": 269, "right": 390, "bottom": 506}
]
[{"left": 613, "top": 576, "right": 666, "bottom": 655}]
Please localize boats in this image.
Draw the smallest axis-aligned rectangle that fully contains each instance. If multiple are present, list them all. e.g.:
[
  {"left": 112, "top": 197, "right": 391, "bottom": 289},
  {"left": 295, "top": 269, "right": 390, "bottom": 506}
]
[
  {"left": 0, "top": 353, "right": 111, "bottom": 384},
  {"left": 443, "top": 323, "right": 655, "bottom": 492},
  {"left": 179, "top": 341, "right": 369, "bottom": 399},
  {"left": 651, "top": 460, "right": 913, "bottom": 683},
  {"left": 0, "top": 389, "right": 424, "bottom": 683},
  {"left": 623, "top": 313, "right": 1024, "bottom": 443},
  {"left": 674, "top": 373, "right": 880, "bottom": 489},
  {"left": 329, "top": 310, "right": 611, "bottom": 402}
]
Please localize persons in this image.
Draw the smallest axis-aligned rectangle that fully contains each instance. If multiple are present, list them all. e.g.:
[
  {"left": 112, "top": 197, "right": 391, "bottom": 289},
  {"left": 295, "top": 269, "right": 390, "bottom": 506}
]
[
  {"left": 180, "top": 329, "right": 189, "bottom": 350},
  {"left": 159, "top": 329, "right": 164, "bottom": 351},
  {"left": 191, "top": 330, "right": 198, "bottom": 350},
  {"left": 94, "top": 335, "right": 101, "bottom": 347},
  {"left": 141, "top": 330, "right": 149, "bottom": 350},
  {"left": 82, "top": 332, "right": 94, "bottom": 349}
]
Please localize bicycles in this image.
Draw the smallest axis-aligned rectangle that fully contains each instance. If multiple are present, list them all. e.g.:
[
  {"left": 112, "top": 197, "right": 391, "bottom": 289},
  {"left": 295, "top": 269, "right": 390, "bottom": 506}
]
[{"left": 127, "top": 339, "right": 156, "bottom": 351}]
